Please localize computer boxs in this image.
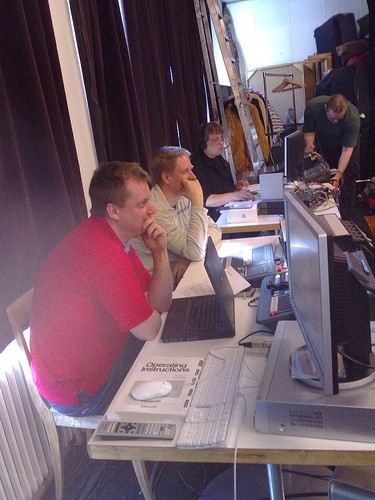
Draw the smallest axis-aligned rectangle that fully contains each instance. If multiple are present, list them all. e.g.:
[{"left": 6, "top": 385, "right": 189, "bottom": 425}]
[
  {"left": 255, "top": 321, "right": 375, "bottom": 442},
  {"left": 258, "top": 172, "right": 283, "bottom": 200}
]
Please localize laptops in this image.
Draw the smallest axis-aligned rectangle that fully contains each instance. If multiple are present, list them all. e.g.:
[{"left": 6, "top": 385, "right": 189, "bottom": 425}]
[{"left": 160, "top": 236, "right": 235, "bottom": 343}]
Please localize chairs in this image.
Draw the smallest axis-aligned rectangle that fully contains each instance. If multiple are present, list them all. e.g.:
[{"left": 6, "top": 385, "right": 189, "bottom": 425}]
[{"left": 6, "top": 287, "right": 157, "bottom": 500}]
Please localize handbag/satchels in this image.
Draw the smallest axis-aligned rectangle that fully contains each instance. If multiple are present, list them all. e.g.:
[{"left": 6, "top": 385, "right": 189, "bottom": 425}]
[{"left": 303, "top": 154, "right": 330, "bottom": 182}]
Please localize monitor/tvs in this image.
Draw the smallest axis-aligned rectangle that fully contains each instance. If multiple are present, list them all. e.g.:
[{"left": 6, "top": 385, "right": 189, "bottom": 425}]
[
  {"left": 281, "top": 192, "right": 375, "bottom": 396},
  {"left": 283, "top": 129, "right": 304, "bottom": 183},
  {"left": 317, "top": 214, "right": 373, "bottom": 264}
]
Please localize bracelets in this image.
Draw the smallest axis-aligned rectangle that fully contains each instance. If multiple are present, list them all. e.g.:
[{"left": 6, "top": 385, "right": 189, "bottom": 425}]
[{"left": 337, "top": 171, "right": 342, "bottom": 175}]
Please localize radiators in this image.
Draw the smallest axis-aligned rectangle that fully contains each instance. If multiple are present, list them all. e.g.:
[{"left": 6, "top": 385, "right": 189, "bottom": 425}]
[{"left": 0, "top": 360, "right": 73, "bottom": 500}]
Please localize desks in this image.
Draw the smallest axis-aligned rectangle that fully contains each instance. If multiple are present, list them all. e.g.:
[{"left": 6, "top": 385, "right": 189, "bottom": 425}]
[
  {"left": 87, "top": 238, "right": 375, "bottom": 500},
  {"left": 216, "top": 183, "right": 342, "bottom": 235}
]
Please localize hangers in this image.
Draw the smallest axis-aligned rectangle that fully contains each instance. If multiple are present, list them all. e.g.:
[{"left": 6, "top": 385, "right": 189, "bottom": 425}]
[{"left": 272, "top": 75, "right": 307, "bottom": 93}]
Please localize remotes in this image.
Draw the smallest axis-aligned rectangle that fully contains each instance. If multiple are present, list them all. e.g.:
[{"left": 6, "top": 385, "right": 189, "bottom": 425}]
[{"left": 97, "top": 420, "right": 176, "bottom": 440}]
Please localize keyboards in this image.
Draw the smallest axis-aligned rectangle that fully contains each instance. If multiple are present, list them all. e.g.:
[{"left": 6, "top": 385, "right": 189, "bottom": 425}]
[{"left": 176, "top": 344, "right": 244, "bottom": 449}]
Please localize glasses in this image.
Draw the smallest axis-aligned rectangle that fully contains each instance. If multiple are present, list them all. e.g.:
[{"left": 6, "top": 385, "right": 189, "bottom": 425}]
[{"left": 207, "top": 136, "right": 225, "bottom": 144}]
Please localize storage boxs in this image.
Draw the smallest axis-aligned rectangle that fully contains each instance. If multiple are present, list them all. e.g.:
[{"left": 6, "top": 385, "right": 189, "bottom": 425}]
[{"left": 229, "top": 204, "right": 258, "bottom": 223}]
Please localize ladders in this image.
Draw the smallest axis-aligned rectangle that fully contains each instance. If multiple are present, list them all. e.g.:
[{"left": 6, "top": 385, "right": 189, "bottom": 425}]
[{"left": 194, "top": 0, "right": 266, "bottom": 186}]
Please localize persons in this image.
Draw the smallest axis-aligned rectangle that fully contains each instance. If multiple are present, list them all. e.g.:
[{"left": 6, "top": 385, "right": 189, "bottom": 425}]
[
  {"left": 302, "top": 94, "right": 360, "bottom": 220},
  {"left": 126, "top": 146, "right": 222, "bottom": 287},
  {"left": 193, "top": 122, "right": 255, "bottom": 221},
  {"left": 31, "top": 162, "right": 211, "bottom": 492}
]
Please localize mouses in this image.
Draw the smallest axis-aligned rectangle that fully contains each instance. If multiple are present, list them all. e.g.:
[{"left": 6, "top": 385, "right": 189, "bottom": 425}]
[{"left": 131, "top": 380, "right": 171, "bottom": 400}]
